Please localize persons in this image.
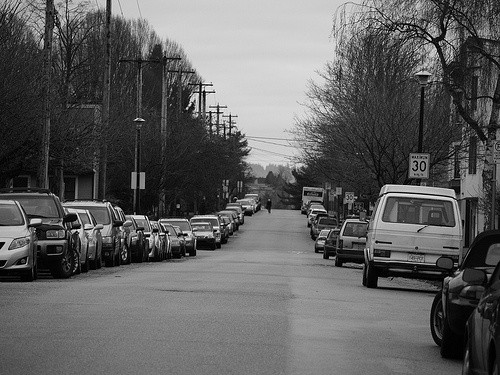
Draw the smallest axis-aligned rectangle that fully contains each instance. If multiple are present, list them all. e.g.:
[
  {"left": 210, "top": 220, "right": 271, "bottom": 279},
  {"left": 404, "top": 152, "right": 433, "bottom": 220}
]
[{"left": 267, "top": 198, "right": 272, "bottom": 213}]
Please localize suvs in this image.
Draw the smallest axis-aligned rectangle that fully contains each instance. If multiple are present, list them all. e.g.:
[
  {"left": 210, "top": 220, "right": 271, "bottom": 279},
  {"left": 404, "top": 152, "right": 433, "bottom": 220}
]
[
  {"left": 0, "top": 187, "right": 80, "bottom": 279},
  {"left": 158, "top": 218, "right": 197, "bottom": 256}
]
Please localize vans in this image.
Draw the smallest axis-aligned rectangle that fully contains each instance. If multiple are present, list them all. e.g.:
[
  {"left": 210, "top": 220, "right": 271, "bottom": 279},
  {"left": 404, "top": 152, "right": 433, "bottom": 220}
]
[{"left": 359, "top": 184, "right": 463, "bottom": 288}]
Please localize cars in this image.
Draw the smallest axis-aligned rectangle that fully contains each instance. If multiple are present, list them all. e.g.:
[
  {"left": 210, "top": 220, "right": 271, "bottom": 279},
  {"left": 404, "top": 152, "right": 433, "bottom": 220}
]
[
  {"left": 461, "top": 260, "right": 500, "bottom": 375},
  {"left": 335, "top": 219, "right": 369, "bottom": 267},
  {"left": 60, "top": 198, "right": 186, "bottom": 273},
  {"left": 315, "top": 230, "right": 331, "bottom": 253},
  {"left": 0, "top": 200, "right": 42, "bottom": 282},
  {"left": 304, "top": 200, "right": 339, "bottom": 240},
  {"left": 190, "top": 193, "right": 261, "bottom": 250},
  {"left": 323, "top": 229, "right": 340, "bottom": 260},
  {"left": 430, "top": 229, "right": 500, "bottom": 361}
]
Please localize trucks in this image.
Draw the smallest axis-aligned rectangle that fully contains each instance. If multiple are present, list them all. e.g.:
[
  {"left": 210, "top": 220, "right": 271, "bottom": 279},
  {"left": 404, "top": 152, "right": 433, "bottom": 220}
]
[{"left": 301, "top": 186, "right": 325, "bottom": 214}]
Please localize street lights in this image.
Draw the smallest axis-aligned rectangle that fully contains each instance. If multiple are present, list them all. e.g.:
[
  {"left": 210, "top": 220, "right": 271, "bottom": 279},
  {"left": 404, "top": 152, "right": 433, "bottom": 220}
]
[
  {"left": 354, "top": 146, "right": 365, "bottom": 168},
  {"left": 415, "top": 71, "right": 432, "bottom": 186},
  {"left": 133, "top": 117, "right": 146, "bottom": 215}
]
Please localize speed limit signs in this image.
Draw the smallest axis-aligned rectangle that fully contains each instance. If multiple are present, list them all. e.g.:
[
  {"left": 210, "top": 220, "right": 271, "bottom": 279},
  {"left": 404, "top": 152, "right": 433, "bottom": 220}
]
[
  {"left": 345, "top": 191, "right": 354, "bottom": 204},
  {"left": 409, "top": 152, "right": 430, "bottom": 179}
]
